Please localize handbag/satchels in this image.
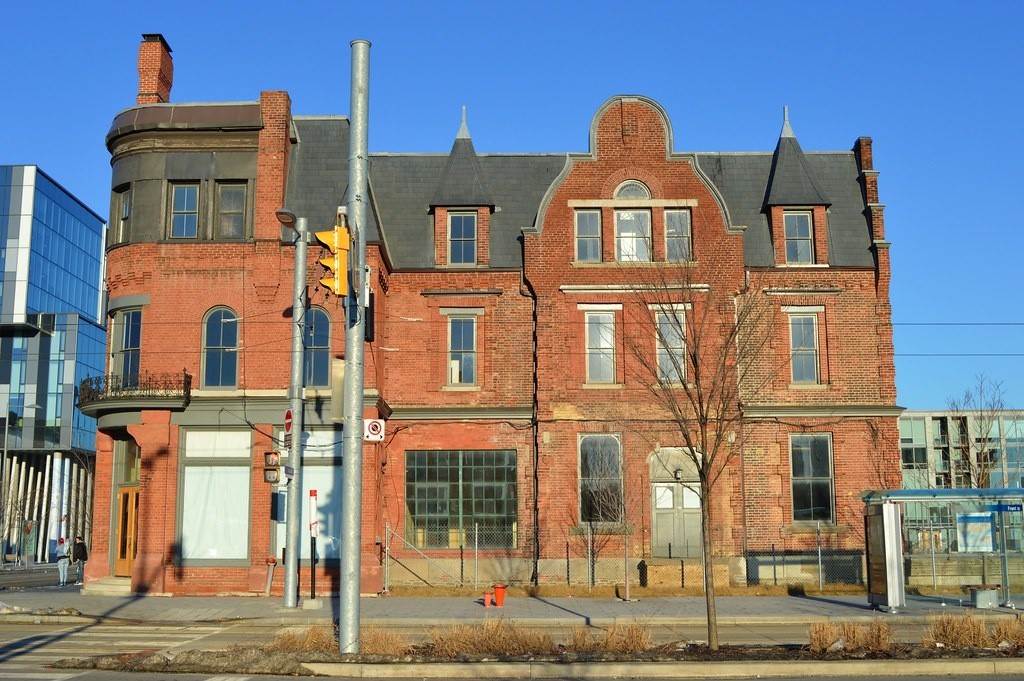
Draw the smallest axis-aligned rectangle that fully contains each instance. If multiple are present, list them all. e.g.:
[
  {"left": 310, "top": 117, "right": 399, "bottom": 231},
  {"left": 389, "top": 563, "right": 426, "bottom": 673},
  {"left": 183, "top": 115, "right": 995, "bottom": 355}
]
[{"left": 69, "top": 559, "right": 72, "bottom": 566}]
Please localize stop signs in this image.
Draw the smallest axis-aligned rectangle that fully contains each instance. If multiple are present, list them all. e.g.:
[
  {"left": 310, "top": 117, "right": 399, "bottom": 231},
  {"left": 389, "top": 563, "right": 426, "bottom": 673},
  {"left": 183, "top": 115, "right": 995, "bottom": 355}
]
[{"left": 284, "top": 409, "right": 292, "bottom": 434}]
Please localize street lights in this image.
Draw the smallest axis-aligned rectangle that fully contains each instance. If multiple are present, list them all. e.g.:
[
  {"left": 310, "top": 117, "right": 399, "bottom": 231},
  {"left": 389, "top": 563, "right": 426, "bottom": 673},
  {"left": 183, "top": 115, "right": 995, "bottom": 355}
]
[{"left": 272, "top": 208, "right": 310, "bottom": 610}]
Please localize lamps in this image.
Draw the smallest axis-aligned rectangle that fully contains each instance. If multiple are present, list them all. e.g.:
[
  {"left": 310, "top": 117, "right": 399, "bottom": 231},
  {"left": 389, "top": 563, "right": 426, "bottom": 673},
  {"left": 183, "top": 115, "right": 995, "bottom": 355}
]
[{"left": 675, "top": 468, "right": 683, "bottom": 479}]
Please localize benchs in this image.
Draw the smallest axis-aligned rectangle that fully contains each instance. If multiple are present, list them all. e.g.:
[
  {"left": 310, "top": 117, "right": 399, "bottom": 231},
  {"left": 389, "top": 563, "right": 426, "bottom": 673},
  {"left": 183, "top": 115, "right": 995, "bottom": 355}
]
[{"left": 960, "top": 584, "right": 1011, "bottom": 609}]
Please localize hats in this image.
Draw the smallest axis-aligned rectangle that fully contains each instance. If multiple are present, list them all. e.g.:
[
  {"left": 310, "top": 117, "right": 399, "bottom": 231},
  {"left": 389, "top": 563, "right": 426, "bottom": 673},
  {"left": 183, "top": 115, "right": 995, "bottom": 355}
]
[{"left": 58, "top": 538, "right": 64, "bottom": 544}]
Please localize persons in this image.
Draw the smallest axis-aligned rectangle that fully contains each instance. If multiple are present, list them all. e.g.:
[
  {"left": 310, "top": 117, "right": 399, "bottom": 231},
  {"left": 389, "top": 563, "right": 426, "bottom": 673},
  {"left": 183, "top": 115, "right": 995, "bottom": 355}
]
[
  {"left": 73, "top": 536, "right": 88, "bottom": 585},
  {"left": 55, "top": 537, "right": 70, "bottom": 586}
]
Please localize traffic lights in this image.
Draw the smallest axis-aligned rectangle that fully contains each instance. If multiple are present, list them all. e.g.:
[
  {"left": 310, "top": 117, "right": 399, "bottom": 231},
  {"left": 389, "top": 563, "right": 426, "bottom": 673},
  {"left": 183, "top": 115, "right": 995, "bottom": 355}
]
[
  {"left": 314, "top": 225, "right": 349, "bottom": 296},
  {"left": 264, "top": 451, "right": 283, "bottom": 485}
]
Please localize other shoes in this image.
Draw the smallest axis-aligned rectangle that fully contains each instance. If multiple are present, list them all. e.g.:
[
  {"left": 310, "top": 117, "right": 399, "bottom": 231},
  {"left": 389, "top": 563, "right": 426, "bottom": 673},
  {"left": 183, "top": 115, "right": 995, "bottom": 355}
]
[
  {"left": 64, "top": 582, "right": 67, "bottom": 586},
  {"left": 58, "top": 583, "right": 63, "bottom": 587},
  {"left": 74, "top": 581, "right": 83, "bottom": 586}
]
